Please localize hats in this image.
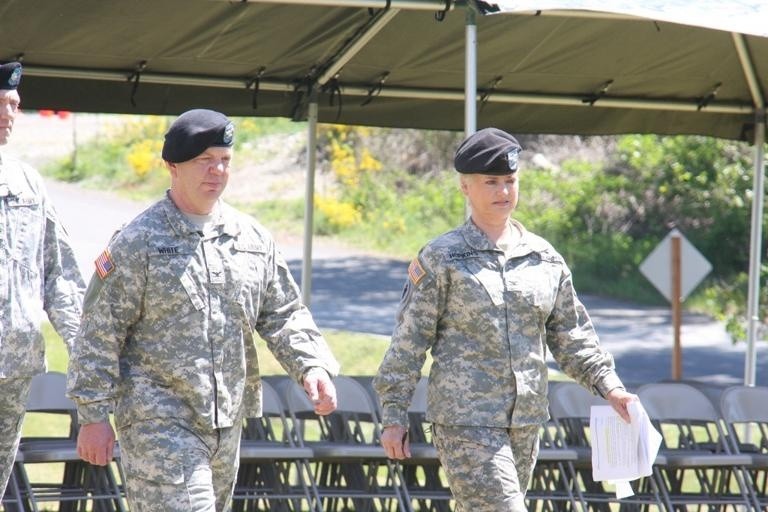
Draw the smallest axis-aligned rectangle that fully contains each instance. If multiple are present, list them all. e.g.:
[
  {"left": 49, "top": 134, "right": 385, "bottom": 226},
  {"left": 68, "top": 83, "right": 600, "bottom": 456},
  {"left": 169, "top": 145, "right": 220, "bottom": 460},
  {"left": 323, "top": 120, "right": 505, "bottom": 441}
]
[
  {"left": 0, "top": 62, "right": 23, "bottom": 90},
  {"left": 454, "top": 128, "right": 523, "bottom": 174},
  {"left": 162, "top": 109, "right": 234, "bottom": 162}
]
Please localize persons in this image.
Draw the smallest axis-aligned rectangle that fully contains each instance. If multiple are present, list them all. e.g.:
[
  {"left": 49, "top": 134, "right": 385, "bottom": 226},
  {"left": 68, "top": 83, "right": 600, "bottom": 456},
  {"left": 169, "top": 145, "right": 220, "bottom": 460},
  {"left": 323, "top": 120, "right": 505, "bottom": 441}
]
[
  {"left": 370, "top": 127, "right": 639, "bottom": 511},
  {"left": 0, "top": 61, "right": 89, "bottom": 512},
  {"left": 64, "top": 108, "right": 340, "bottom": 511}
]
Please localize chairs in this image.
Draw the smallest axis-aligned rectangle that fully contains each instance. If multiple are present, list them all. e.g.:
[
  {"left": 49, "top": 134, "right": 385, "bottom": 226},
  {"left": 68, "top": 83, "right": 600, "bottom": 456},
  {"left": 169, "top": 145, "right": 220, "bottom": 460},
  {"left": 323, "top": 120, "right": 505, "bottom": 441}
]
[{"left": 1, "top": 368, "right": 767, "bottom": 512}]
